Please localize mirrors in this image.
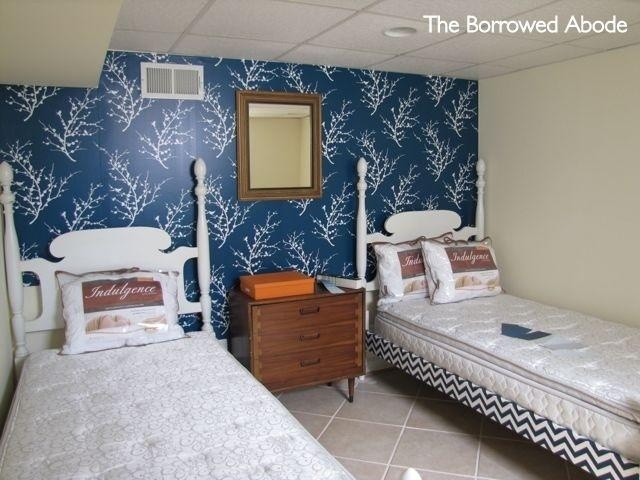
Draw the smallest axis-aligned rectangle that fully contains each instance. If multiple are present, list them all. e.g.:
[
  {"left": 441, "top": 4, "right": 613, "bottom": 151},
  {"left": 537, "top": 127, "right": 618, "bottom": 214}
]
[{"left": 237, "top": 90, "right": 321, "bottom": 201}]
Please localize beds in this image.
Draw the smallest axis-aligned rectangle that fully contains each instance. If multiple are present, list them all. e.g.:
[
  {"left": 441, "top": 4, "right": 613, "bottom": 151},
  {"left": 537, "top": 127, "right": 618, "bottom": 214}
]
[
  {"left": 0, "top": 157, "right": 359, "bottom": 480},
  {"left": 355, "top": 157, "right": 640, "bottom": 480}
]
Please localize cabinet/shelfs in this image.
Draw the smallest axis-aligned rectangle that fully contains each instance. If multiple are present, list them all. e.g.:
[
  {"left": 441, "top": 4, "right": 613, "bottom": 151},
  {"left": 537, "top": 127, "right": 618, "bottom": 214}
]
[{"left": 228, "top": 284, "right": 365, "bottom": 403}]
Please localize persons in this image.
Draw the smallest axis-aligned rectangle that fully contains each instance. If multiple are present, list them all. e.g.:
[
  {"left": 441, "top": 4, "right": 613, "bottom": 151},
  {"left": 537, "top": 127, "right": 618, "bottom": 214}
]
[
  {"left": 454, "top": 274, "right": 502, "bottom": 290},
  {"left": 404, "top": 278, "right": 428, "bottom": 293},
  {"left": 85, "top": 314, "right": 166, "bottom": 334}
]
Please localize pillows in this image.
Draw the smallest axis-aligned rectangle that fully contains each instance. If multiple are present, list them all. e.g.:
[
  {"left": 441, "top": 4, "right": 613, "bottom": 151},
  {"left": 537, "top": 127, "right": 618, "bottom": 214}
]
[
  {"left": 369, "top": 231, "right": 506, "bottom": 307},
  {"left": 55, "top": 269, "right": 191, "bottom": 356}
]
